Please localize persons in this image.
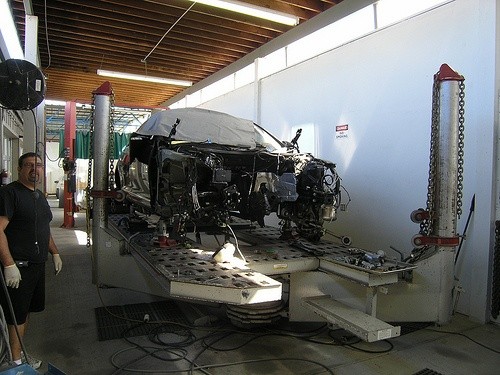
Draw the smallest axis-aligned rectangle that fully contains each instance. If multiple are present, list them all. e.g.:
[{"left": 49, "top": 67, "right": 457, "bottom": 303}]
[{"left": 0, "top": 152, "right": 62, "bottom": 371}]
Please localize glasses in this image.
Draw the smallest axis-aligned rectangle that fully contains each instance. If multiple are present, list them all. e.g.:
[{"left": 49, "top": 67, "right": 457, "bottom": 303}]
[{"left": 21, "top": 163, "right": 44, "bottom": 169}]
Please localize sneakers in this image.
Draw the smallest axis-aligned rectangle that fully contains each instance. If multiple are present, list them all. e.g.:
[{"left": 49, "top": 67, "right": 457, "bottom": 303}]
[{"left": 10, "top": 354, "right": 43, "bottom": 370}]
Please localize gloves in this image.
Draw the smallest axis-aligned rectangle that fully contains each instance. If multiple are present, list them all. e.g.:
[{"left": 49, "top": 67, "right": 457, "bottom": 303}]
[
  {"left": 4, "top": 264, "right": 22, "bottom": 288},
  {"left": 52, "top": 254, "right": 63, "bottom": 276}
]
